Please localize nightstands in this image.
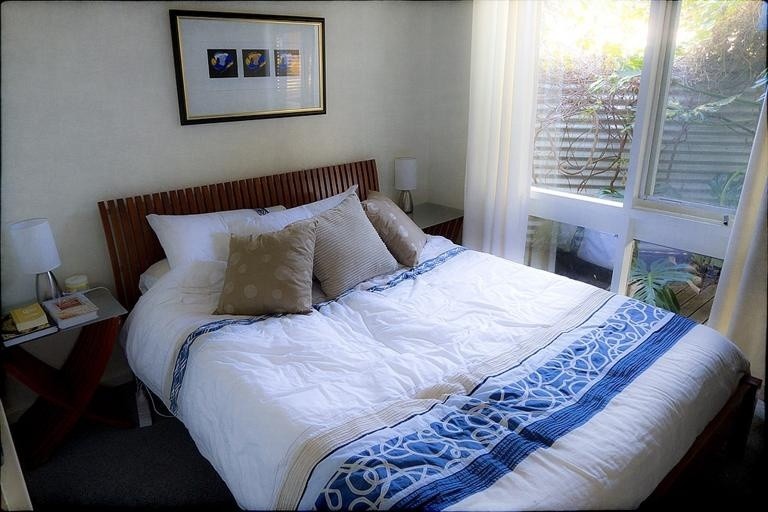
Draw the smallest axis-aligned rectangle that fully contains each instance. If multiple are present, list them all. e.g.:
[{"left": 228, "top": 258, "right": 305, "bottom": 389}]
[
  {"left": 405, "top": 202, "right": 464, "bottom": 244},
  {"left": 1, "top": 286, "right": 129, "bottom": 452}
]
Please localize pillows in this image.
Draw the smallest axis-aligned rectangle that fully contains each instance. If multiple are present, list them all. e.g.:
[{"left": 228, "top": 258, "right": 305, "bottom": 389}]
[
  {"left": 146, "top": 204, "right": 287, "bottom": 269},
  {"left": 361, "top": 189, "right": 427, "bottom": 268},
  {"left": 216, "top": 218, "right": 319, "bottom": 315},
  {"left": 284, "top": 194, "right": 399, "bottom": 300},
  {"left": 218, "top": 184, "right": 359, "bottom": 235}
]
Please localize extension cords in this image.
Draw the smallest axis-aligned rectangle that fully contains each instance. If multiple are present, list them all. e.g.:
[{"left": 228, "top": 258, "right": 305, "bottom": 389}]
[{"left": 135, "top": 391, "right": 153, "bottom": 428}]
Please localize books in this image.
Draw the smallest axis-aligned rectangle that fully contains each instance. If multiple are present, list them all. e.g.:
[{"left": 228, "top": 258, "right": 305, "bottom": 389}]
[{"left": 0, "top": 290, "right": 100, "bottom": 346}]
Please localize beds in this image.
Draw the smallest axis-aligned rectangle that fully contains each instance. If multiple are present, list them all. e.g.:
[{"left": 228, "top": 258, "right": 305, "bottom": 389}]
[{"left": 98, "top": 158, "right": 763, "bottom": 512}]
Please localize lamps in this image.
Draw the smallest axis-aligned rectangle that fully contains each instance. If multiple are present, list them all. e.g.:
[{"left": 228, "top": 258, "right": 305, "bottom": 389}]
[
  {"left": 394, "top": 159, "right": 417, "bottom": 214},
  {"left": 9, "top": 218, "right": 62, "bottom": 302}
]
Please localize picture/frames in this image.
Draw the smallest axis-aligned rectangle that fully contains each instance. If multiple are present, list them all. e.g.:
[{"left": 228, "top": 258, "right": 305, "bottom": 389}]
[{"left": 169, "top": 8, "right": 326, "bottom": 126}]
